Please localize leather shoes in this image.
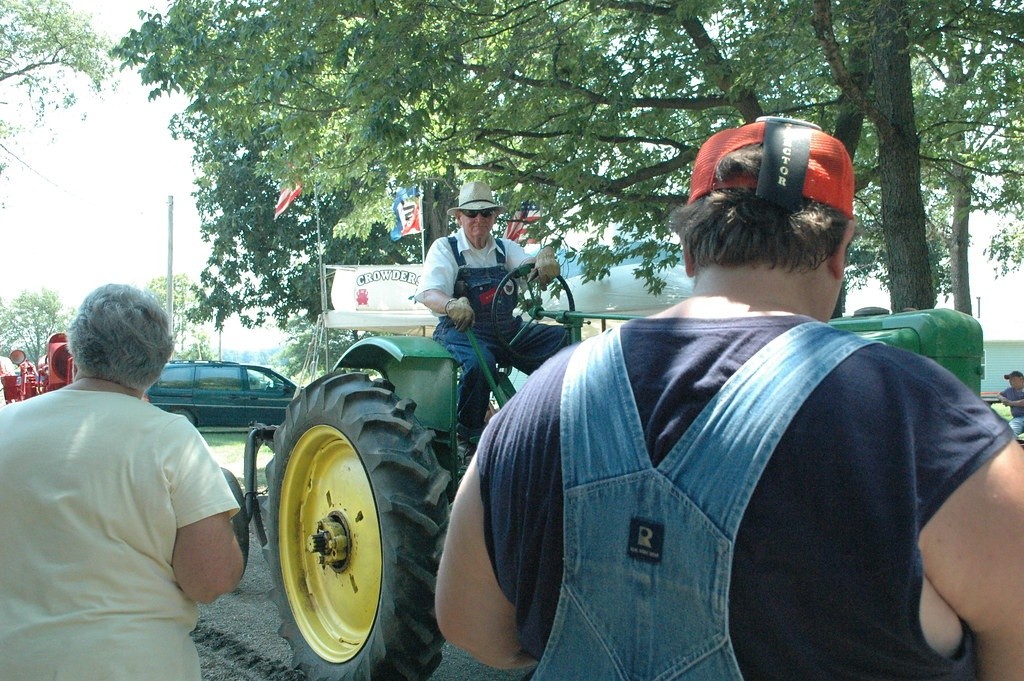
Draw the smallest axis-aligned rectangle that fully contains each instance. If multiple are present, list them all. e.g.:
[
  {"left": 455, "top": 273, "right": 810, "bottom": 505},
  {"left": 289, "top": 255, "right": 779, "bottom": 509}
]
[{"left": 456, "top": 434, "right": 476, "bottom": 456}]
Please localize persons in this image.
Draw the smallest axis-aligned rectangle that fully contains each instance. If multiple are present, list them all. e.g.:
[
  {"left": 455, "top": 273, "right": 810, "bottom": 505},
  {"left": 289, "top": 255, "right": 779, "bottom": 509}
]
[
  {"left": 1, "top": 281, "right": 245, "bottom": 681},
  {"left": 994, "top": 368, "right": 1024, "bottom": 438},
  {"left": 437, "top": 111, "right": 1024, "bottom": 681},
  {"left": 414, "top": 174, "right": 574, "bottom": 469}
]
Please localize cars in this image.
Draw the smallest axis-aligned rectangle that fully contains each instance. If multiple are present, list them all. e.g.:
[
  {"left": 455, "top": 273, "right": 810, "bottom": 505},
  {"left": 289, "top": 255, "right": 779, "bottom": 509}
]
[{"left": 142, "top": 360, "right": 307, "bottom": 429}]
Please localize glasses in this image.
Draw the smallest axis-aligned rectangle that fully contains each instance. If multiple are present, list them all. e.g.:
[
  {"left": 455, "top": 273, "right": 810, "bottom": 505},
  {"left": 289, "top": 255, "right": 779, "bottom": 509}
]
[{"left": 457, "top": 208, "right": 497, "bottom": 218}]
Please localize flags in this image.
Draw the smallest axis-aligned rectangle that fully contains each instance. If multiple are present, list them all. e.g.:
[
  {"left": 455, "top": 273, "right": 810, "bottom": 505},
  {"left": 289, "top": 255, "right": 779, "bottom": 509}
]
[
  {"left": 269, "top": 135, "right": 316, "bottom": 220},
  {"left": 389, "top": 185, "right": 427, "bottom": 242},
  {"left": 499, "top": 186, "right": 549, "bottom": 247}
]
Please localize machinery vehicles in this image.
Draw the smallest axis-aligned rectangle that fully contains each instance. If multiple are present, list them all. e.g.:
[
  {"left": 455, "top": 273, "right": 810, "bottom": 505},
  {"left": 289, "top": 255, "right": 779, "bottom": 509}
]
[{"left": 261, "top": 263, "right": 987, "bottom": 680}]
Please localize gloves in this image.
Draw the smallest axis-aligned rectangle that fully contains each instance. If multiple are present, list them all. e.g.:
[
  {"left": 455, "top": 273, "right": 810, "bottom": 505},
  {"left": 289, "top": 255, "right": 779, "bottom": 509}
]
[
  {"left": 446, "top": 296, "right": 476, "bottom": 333},
  {"left": 535, "top": 246, "right": 560, "bottom": 292}
]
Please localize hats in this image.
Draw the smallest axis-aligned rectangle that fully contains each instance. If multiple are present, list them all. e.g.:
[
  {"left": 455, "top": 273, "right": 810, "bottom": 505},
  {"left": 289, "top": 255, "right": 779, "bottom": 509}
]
[
  {"left": 447, "top": 180, "right": 506, "bottom": 216},
  {"left": 1004, "top": 371, "right": 1023, "bottom": 379},
  {"left": 687, "top": 115, "right": 856, "bottom": 220}
]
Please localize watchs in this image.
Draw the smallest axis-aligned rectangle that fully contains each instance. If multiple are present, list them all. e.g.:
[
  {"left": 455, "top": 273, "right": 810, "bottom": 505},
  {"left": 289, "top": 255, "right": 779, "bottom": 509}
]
[{"left": 444, "top": 295, "right": 459, "bottom": 316}]
